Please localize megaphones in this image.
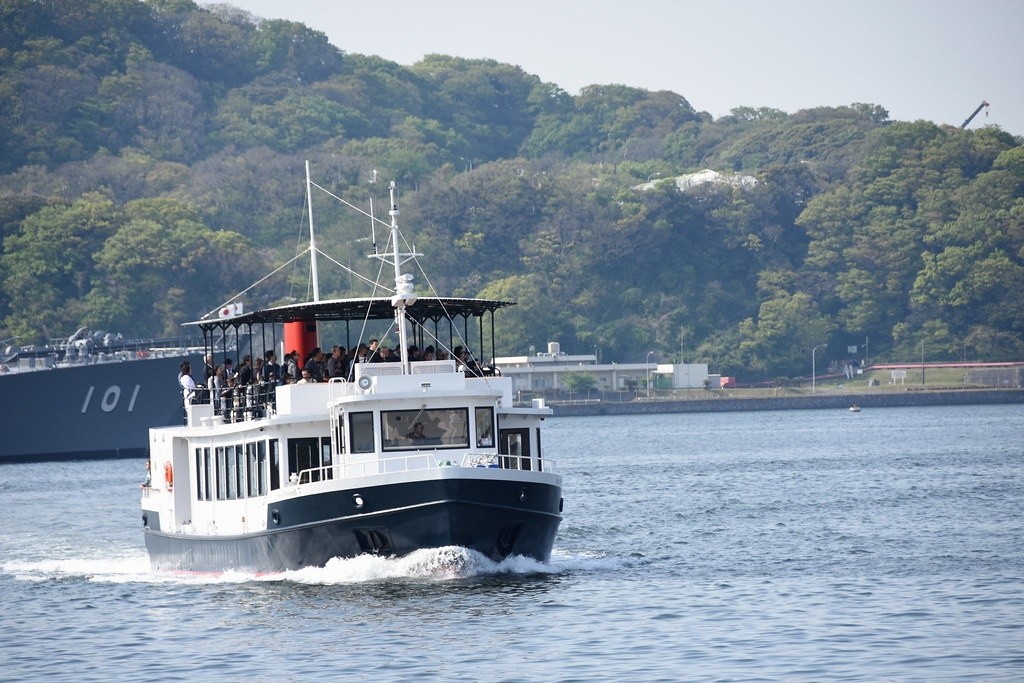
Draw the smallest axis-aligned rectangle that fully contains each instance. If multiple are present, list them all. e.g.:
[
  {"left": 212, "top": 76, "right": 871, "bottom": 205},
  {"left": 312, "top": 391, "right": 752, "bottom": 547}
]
[{"left": 358, "top": 375, "right": 372, "bottom": 390}]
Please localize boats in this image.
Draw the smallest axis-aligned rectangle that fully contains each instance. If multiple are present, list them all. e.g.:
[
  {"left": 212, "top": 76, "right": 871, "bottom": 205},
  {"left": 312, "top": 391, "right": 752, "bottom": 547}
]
[
  {"left": 139, "top": 160, "right": 565, "bottom": 580},
  {"left": 0, "top": 322, "right": 254, "bottom": 464}
]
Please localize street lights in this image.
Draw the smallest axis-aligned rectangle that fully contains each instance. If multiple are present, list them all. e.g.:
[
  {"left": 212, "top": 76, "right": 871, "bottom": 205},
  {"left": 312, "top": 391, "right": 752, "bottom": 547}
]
[
  {"left": 813, "top": 344, "right": 828, "bottom": 394},
  {"left": 646, "top": 351, "right": 653, "bottom": 399}
]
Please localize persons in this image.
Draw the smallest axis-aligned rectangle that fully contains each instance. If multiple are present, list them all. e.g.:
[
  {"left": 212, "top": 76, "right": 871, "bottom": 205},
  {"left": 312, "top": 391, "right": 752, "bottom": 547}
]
[
  {"left": 405, "top": 423, "right": 428, "bottom": 443},
  {"left": 175, "top": 339, "right": 489, "bottom": 414}
]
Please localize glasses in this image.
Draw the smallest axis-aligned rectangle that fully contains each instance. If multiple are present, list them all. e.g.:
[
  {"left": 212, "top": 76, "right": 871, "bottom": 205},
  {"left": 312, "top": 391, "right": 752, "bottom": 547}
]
[{"left": 296, "top": 354, "right": 300, "bottom": 356}]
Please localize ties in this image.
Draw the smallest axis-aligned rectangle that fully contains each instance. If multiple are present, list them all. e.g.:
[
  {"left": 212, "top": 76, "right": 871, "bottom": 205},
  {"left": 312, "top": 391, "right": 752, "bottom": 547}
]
[{"left": 228, "top": 368, "right": 231, "bottom": 376}]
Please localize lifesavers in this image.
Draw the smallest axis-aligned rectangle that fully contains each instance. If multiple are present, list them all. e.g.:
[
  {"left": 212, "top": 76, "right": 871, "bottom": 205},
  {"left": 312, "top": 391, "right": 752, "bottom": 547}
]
[{"left": 164, "top": 461, "right": 173, "bottom": 493}]
